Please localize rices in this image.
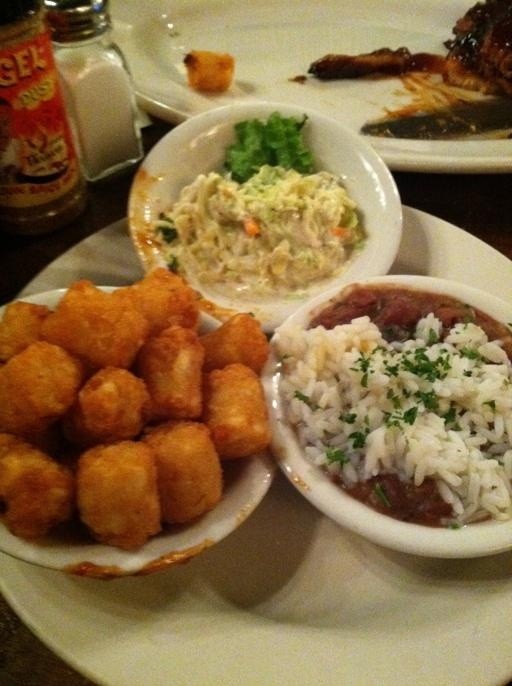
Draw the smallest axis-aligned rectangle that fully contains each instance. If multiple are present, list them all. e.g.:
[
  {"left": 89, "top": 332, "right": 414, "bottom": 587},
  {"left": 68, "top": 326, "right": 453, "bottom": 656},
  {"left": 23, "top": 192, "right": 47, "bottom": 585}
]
[{"left": 271, "top": 312, "right": 512, "bottom": 529}]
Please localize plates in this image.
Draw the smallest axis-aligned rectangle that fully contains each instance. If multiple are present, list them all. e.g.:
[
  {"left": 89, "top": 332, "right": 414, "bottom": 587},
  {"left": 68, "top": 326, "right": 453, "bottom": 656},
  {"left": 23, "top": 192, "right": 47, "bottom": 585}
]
[
  {"left": 126, "top": 99, "right": 404, "bottom": 333},
  {"left": 262, "top": 274, "right": 512, "bottom": 560},
  {"left": 0, "top": 287, "right": 279, "bottom": 578},
  {"left": 105, "top": 0, "right": 512, "bottom": 174},
  {"left": 0, "top": 201, "right": 512, "bottom": 686}
]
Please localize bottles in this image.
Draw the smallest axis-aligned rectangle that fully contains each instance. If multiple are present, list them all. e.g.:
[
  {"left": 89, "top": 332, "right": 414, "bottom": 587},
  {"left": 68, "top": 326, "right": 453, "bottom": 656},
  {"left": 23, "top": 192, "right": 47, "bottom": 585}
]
[
  {"left": 0, "top": 0, "right": 89, "bottom": 239},
  {"left": 43, "top": 0, "right": 146, "bottom": 181}
]
